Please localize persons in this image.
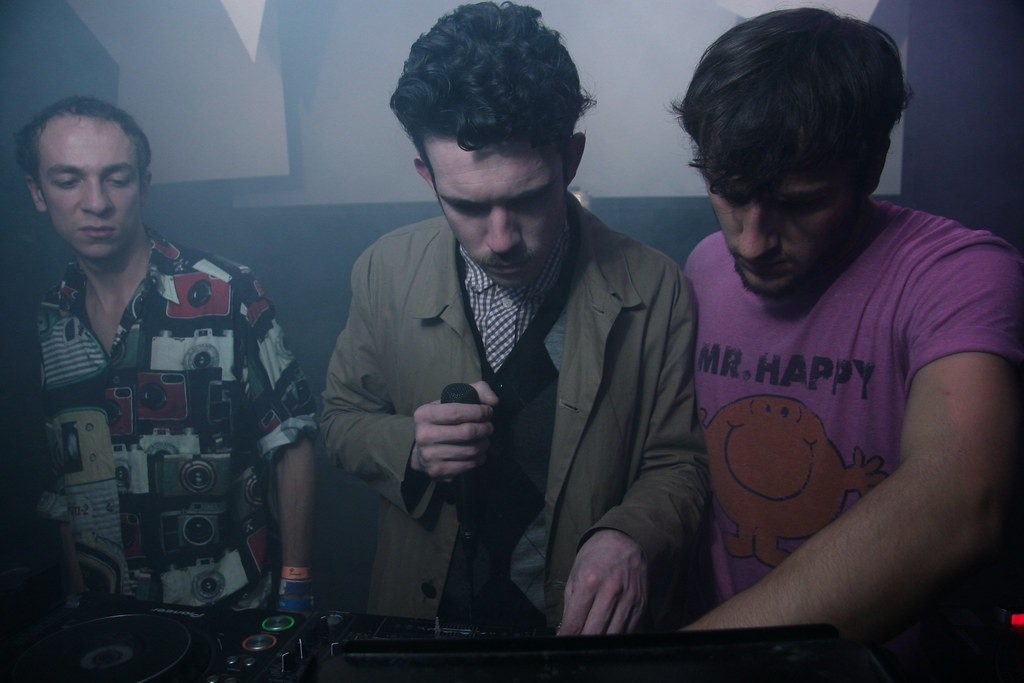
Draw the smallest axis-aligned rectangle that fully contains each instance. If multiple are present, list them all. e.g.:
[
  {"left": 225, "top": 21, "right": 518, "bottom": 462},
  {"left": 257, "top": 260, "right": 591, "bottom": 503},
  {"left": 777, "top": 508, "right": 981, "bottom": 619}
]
[
  {"left": 322, "top": 0, "right": 709, "bottom": 637},
  {"left": 681, "top": 6, "right": 1024, "bottom": 629},
  {"left": 22, "top": 96, "right": 319, "bottom": 617}
]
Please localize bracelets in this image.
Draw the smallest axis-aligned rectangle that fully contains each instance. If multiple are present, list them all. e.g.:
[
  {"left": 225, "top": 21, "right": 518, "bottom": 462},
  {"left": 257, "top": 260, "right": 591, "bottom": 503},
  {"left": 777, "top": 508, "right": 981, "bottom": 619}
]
[
  {"left": 282, "top": 565, "right": 313, "bottom": 579},
  {"left": 279, "top": 578, "right": 314, "bottom": 596},
  {"left": 277, "top": 598, "right": 313, "bottom": 614}
]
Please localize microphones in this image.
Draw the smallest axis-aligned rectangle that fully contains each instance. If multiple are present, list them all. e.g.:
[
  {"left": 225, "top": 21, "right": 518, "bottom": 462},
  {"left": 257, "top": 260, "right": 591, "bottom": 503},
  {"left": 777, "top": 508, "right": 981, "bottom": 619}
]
[{"left": 440, "top": 382, "right": 478, "bottom": 560}]
